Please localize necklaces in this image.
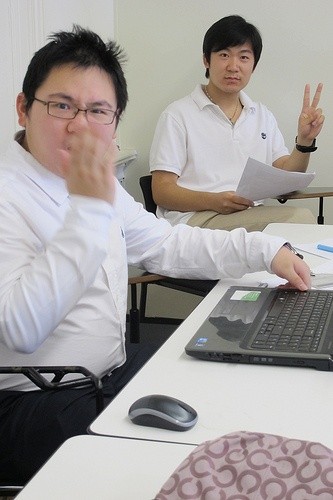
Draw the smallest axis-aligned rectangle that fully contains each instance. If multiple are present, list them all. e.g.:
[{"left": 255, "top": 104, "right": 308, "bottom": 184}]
[{"left": 204, "top": 85, "right": 239, "bottom": 121}]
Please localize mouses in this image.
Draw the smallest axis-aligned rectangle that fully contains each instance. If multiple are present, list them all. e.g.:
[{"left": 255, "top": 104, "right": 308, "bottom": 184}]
[{"left": 129, "top": 395, "right": 198, "bottom": 430}]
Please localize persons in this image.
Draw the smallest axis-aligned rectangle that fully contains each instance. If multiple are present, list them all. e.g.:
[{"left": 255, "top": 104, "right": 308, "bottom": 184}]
[
  {"left": 150, "top": 16, "right": 325, "bottom": 233},
  {"left": 0, "top": 26, "right": 310, "bottom": 487}
]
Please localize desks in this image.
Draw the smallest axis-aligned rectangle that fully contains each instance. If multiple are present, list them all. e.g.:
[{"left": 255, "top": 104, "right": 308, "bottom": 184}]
[{"left": 16, "top": 224, "right": 333, "bottom": 500}]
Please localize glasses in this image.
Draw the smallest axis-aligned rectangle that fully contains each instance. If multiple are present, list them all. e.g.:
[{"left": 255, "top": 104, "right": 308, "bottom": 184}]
[{"left": 34, "top": 96, "right": 118, "bottom": 125}]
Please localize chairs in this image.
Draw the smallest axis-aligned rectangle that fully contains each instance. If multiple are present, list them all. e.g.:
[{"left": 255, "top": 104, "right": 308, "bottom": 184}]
[
  {"left": 0, "top": 275, "right": 165, "bottom": 492},
  {"left": 138, "top": 173, "right": 218, "bottom": 317}
]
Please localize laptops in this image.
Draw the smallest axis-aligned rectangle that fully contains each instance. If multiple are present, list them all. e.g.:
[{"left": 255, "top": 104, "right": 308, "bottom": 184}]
[{"left": 186, "top": 284, "right": 332, "bottom": 371}]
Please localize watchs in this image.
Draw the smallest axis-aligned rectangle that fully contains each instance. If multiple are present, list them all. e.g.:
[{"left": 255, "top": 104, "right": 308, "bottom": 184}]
[{"left": 295, "top": 135, "right": 318, "bottom": 154}]
